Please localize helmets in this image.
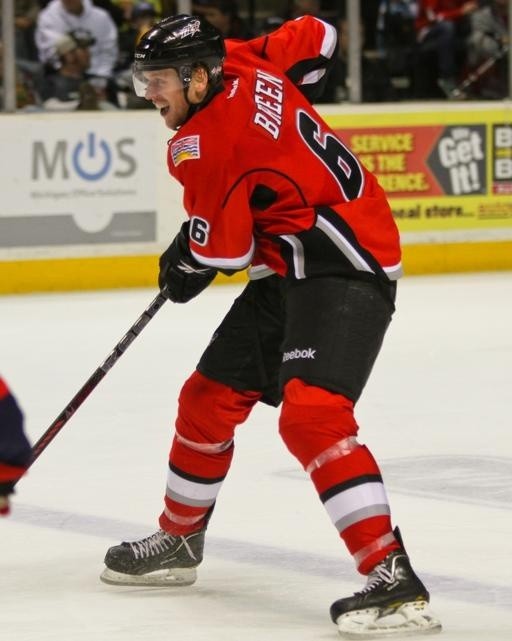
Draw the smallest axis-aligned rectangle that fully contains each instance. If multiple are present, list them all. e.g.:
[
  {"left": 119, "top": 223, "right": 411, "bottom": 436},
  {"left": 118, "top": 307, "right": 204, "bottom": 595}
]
[{"left": 131, "top": 12, "right": 226, "bottom": 99}]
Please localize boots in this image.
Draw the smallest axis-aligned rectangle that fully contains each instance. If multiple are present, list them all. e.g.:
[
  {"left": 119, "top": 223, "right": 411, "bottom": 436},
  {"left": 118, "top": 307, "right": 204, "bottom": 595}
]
[
  {"left": 104, "top": 503, "right": 216, "bottom": 576},
  {"left": 329, "top": 524, "right": 430, "bottom": 626}
]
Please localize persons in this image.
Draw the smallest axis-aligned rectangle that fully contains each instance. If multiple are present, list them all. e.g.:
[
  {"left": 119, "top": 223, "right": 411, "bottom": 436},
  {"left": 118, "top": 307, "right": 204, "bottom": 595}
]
[{"left": 104, "top": 15, "right": 430, "bottom": 631}]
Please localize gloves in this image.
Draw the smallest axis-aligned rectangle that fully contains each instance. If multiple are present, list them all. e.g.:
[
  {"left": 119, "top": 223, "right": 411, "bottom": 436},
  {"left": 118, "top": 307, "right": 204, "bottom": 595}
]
[{"left": 157, "top": 221, "right": 219, "bottom": 304}]
